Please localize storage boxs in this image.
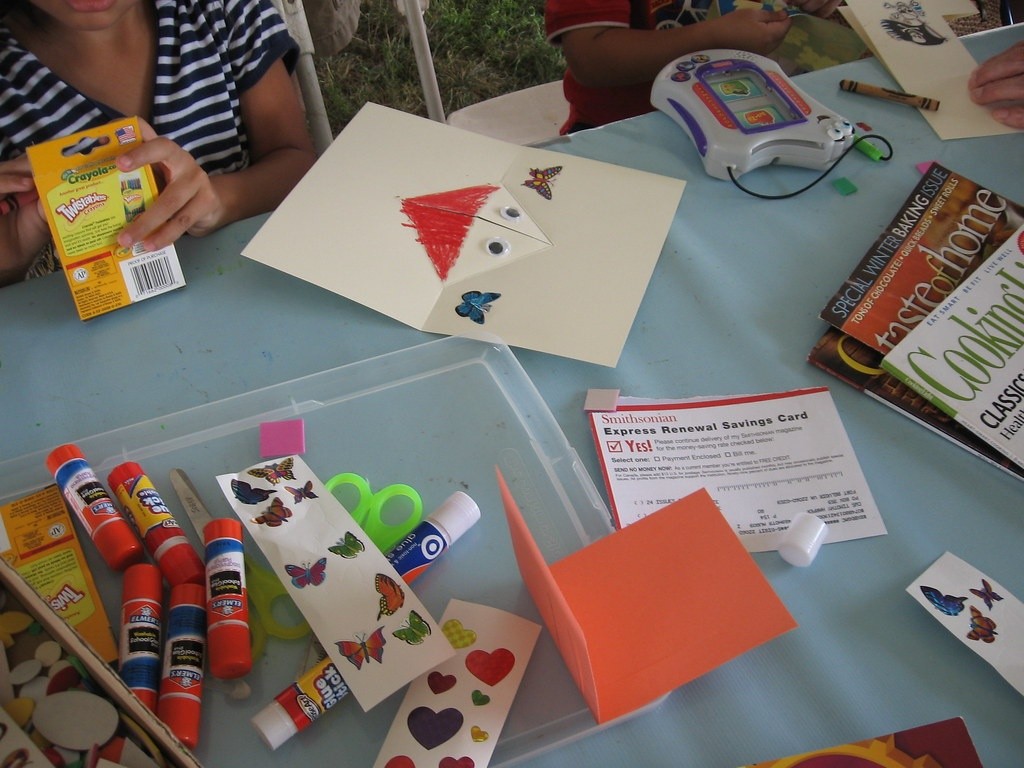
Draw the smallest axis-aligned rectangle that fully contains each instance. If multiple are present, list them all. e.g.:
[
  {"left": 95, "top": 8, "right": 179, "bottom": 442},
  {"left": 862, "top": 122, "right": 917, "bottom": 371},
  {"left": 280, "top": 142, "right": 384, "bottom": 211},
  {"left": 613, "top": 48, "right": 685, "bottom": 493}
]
[{"left": 24, "top": 115, "right": 187, "bottom": 320}]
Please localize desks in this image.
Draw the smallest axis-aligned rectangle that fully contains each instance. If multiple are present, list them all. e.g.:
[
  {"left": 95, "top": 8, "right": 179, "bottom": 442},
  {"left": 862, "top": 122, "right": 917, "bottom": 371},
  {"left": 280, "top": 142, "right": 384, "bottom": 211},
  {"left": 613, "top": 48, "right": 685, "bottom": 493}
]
[{"left": 0, "top": 20, "right": 1024, "bottom": 768}]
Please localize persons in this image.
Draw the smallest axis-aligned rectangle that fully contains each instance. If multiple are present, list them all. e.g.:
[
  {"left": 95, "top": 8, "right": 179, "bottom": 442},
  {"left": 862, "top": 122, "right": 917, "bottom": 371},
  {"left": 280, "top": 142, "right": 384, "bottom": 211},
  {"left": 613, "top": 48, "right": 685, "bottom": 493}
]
[
  {"left": 967, "top": 38, "right": 1024, "bottom": 130},
  {"left": 0, "top": 0, "right": 318, "bottom": 282},
  {"left": 543, "top": 0, "right": 843, "bottom": 137}
]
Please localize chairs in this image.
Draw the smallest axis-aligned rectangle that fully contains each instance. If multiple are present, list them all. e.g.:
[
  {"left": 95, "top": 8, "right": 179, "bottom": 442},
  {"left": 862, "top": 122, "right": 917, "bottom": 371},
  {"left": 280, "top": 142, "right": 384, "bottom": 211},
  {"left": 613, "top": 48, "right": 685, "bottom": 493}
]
[{"left": 393, "top": 0, "right": 571, "bottom": 145}]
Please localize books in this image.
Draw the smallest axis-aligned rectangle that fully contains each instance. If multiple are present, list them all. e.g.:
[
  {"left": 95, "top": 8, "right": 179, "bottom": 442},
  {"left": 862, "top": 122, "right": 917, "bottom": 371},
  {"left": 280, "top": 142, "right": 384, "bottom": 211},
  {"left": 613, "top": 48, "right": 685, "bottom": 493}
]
[{"left": 804, "top": 160, "right": 1023, "bottom": 484}]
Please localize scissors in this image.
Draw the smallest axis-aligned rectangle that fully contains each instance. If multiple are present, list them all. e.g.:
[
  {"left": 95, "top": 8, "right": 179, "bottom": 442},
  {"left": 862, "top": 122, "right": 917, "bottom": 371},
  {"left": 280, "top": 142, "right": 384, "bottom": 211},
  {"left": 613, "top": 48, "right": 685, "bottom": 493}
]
[
  {"left": 163, "top": 460, "right": 315, "bottom": 660},
  {"left": 296, "top": 469, "right": 424, "bottom": 680}
]
